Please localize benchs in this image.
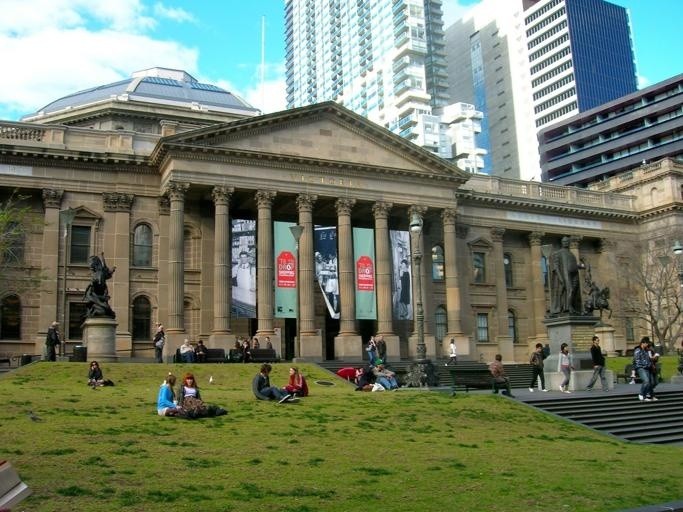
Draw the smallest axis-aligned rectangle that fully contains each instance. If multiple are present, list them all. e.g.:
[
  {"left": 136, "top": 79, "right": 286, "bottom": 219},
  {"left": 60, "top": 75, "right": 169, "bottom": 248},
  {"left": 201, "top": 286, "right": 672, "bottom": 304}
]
[
  {"left": 448, "top": 368, "right": 511, "bottom": 396},
  {"left": 228, "top": 348, "right": 279, "bottom": 363},
  {"left": 172, "top": 347, "right": 226, "bottom": 363},
  {"left": 615, "top": 362, "right": 664, "bottom": 383}
]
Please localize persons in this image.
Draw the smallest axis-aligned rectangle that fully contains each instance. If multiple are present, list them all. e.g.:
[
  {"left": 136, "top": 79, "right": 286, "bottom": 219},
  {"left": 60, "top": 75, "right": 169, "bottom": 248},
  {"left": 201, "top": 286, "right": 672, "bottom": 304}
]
[
  {"left": 44, "top": 320, "right": 62, "bottom": 361},
  {"left": 550, "top": 235, "right": 582, "bottom": 314},
  {"left": 232, "top": 250, "right": 256, "bottom": 292},
  {"left": 87, "top": 360, "right": 105, "bottom": 389},
  {"left": 315, "top": 251, "right": 326, "bottom": 283},
  {"left": 398, "top": 259, "right": 410, "bottom": 317},
  {"left": 325, "top": 250, "right": 340, "bottom": 312}
]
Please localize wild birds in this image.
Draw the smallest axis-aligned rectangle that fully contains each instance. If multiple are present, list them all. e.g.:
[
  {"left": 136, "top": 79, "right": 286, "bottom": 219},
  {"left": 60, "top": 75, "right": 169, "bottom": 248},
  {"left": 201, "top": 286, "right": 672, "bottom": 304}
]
[
  {"left": 92, "top": 384, "right": 96, "bottom": 389},
  {"left": 29, "top": 411, "right": 42, "bottom": 422},
  {"left": 209, "top": 375, "right": 213, "bottom": 383}
]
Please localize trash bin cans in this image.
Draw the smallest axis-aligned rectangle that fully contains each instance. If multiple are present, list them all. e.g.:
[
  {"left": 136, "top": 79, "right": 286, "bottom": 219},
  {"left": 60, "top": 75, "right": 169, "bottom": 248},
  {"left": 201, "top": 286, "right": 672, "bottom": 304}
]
[
  {"left": 72, "top": 346, "right": 87, "bottom": 362},
  {"left": 21, "top": 354, "right": 32, "bottom": 366}
]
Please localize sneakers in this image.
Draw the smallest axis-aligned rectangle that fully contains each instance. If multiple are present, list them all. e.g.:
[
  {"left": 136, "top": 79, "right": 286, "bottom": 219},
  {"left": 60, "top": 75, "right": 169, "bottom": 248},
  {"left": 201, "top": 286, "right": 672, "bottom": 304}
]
[
  {"left": 638, "top": 394, "right": 659, "bottom": 401},
  {"left": 277, "top": 395, "right": 302, "bottom": 404}
]
